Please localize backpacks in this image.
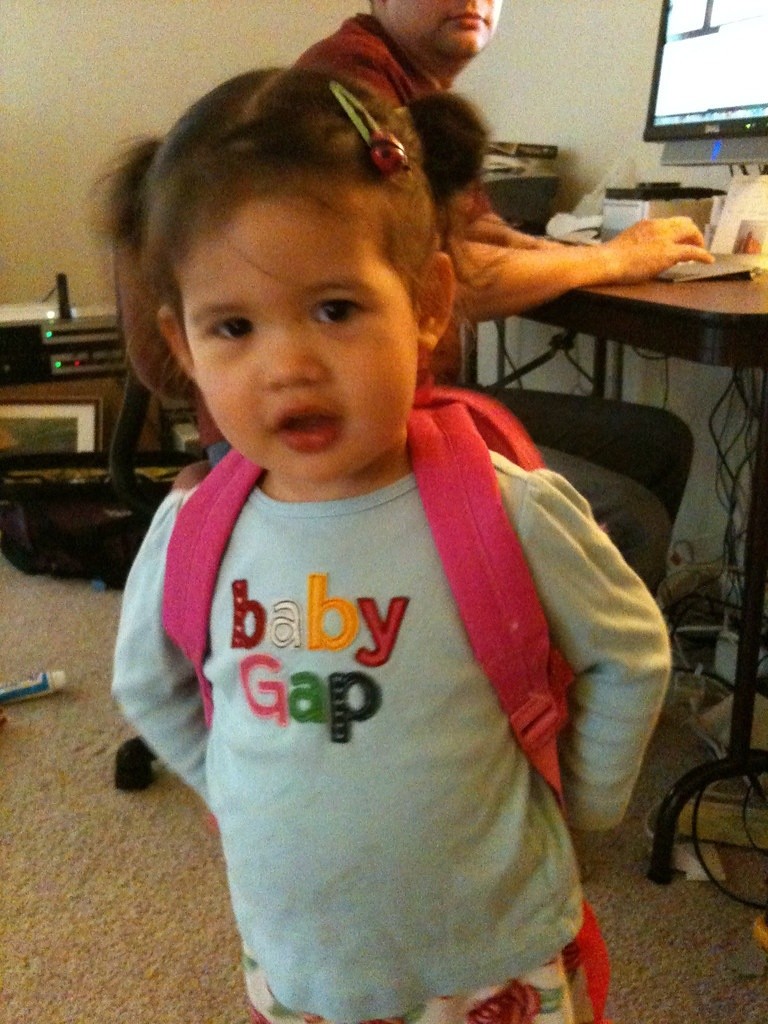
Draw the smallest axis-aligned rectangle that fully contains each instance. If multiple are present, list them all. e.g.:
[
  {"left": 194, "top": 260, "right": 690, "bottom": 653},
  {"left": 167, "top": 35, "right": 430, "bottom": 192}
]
[{"left": 161, "top": 384, "right": 569, "bottom": 810}]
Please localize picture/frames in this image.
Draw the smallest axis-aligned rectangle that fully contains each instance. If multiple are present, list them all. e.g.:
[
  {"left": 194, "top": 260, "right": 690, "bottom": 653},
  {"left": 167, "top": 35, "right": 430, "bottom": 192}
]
[{"left": 0, "top": 395, "right": 105, "bottom": 485}]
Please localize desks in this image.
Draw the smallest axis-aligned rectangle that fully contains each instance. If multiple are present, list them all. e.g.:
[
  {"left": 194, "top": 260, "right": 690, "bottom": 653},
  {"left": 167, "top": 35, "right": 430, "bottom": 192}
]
[{"left": 463, "top": 269, "right": 768, "bottom": 886}]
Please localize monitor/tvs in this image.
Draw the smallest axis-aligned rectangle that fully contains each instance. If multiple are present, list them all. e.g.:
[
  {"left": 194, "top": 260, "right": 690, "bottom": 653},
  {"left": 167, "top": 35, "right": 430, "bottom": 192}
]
[{"left": 641, "top": 0, "right": 767, "bottom": 167}]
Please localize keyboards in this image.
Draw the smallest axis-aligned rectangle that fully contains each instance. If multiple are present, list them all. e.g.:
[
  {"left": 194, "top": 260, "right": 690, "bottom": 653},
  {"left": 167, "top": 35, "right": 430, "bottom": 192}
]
[{"left": 656, "top": 252, "right": 762, "bottom": 284}]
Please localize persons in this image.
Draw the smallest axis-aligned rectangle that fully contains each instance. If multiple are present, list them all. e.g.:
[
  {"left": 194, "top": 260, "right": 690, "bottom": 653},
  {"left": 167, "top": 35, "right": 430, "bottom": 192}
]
[
  {"left": 82, "top": 65, "right": 672, "bottom": 1024},
  {"left": 285, "top": 1, "right": 712, "bottom": 586}
]
